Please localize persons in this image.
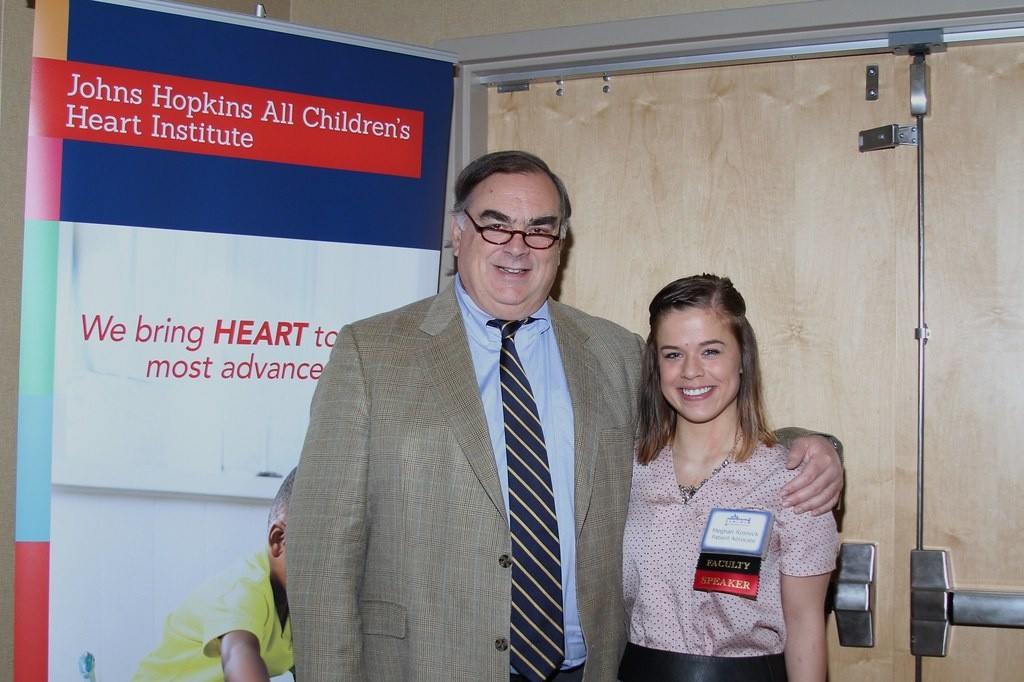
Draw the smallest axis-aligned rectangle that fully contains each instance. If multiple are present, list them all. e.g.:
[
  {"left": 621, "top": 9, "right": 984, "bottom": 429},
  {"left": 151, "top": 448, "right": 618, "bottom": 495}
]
[
  {"left": 129, "top": 466, "right": 297, "bottom": 682},
  {"left": 618, "top": 272, "right": 840, "bottom": 682},
  {"left": 284, "top": 150, "right": 844, "bottom": 682}
]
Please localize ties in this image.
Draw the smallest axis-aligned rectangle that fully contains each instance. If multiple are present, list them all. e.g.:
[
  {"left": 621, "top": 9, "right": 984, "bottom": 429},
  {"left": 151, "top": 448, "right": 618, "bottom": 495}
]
[{"left": 487, "top": 317, "right": 564, "bottom": 682}]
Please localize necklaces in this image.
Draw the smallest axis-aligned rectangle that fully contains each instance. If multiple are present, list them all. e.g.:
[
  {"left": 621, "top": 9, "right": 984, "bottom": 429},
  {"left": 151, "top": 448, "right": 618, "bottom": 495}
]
[{"left": 678, "top": 431, "right": 745, "bottom": 507}]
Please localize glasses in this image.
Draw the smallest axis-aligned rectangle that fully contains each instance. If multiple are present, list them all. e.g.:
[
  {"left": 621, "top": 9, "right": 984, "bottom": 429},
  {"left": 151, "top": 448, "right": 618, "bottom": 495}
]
[{"left": 464, "top": 209, "right": 562, "bottom": 250}]
